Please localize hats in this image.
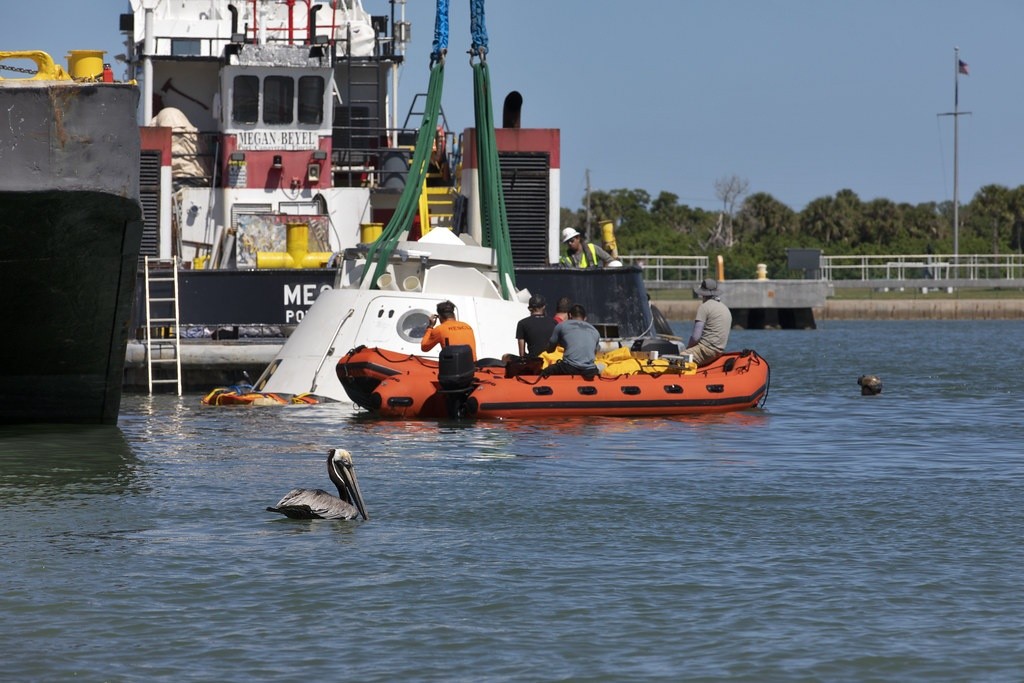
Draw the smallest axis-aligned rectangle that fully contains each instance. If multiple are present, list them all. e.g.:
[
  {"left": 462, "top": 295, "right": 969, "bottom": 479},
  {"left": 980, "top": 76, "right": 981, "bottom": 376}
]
[
  {"left": 694, "top": 278, "right": 724, "bottom": 296},
  {"left": 562, "top": 227, "right": 580, "bottom": 243}
]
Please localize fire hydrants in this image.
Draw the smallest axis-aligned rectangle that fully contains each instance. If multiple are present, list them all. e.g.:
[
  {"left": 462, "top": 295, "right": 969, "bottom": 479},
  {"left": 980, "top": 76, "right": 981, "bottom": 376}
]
[{"left": 756, "top": 263, "right": 770, "bottom": 281}]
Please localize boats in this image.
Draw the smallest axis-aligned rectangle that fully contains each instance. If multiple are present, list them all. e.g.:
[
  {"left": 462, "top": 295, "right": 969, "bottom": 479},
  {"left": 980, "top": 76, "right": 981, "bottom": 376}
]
[
  {"left": 334, "top": 342, "right": 773, "bottom": 418},
  {"left": 0, "top": 37, "right": 142, "bottom": 427}
]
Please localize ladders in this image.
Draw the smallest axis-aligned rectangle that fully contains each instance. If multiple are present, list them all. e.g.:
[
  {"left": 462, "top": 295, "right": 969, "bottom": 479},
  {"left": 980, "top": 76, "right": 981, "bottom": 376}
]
[{"left": 143, "top": 255, "right": 182, "bottom": 398}]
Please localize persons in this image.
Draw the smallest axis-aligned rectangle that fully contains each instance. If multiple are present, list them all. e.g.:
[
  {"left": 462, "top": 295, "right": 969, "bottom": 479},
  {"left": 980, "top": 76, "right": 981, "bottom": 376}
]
[
  {"left": 559, "top": 227, "right": 621, "bottom": 267},
  {"left": 679, "top": 278, "right": 732, "bottom": 369},
  {"left": 421, "top": 302, "right": 477, "bottom": 362},
  {"left": 553, "top": 297, "right": 570, "bottom": 323},
  {"left": 543, "top": 304, "right": 601, "bottom": 375},
  {"left": 516, "top": 294, "right": 558, "bottom": 358}
]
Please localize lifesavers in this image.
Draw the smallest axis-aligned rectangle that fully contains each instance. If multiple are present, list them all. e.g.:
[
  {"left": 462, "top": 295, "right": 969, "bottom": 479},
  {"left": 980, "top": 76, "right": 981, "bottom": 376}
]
[
  {"left": 431, "top": 126, "right": 445, "bottom": 162},
  {"left": 651, "top": 304, "right": 673, "bottom": 335}
]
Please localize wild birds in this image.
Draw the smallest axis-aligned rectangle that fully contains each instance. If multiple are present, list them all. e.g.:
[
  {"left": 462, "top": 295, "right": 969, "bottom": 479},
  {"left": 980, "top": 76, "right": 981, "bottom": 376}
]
[{"left": 263, "top": 448, "right": 368, "bottom": 522}]
